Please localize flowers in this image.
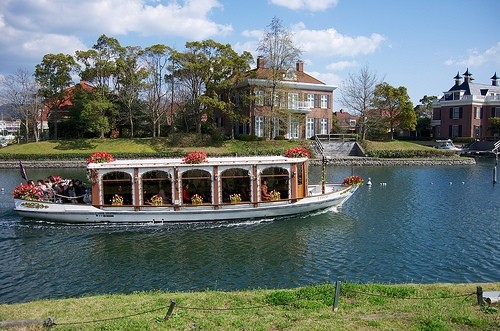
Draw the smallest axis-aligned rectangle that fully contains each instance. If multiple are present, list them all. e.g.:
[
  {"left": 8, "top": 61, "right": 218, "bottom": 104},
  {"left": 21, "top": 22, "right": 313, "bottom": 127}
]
[
  {"left": 268, "top": 189, "right": 281, "bottom": 199},
  {"left": 150, "top": 195, "right": 162, "bottom": 203},
  {"left": 343, "top": 175, "right": 364, "bottom": 186},
  {"left": 11, "top": 175, "right": 70, "bottom": 210},
  {"left": 110, "top": 194, "right": 123, "bottom": 204},
  {"left": 227, "top": 193, "right": 243, "bottom": 201},
  {"left": 180, "top": 150, "right": 208, "bottom": 164},
  {"left": 190, "top": 193, "right": 203, "bottom": 202},
  {"left": 86, "top": 152, "right": 115, "bottom": 164},
  {"left": 285, "top": 146, "right": 310, "bottom": 161}
]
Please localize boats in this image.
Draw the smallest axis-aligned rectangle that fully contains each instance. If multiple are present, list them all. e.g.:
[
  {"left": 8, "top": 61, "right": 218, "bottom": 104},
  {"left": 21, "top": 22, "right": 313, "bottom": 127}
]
[
  {"left": 11, "top": 152, "right": 364, "bottom": 223},
  {"left": 432, "top": 139, "right": 463, "bottom": 153}
]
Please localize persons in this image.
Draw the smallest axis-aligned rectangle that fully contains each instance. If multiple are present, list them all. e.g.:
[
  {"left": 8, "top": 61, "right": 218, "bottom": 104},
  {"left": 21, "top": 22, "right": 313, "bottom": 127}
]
[
  {"left": 183, "top": 183, "right": 190, "bottom": 201},
  {"left": 28, "top": 176, "right": 86, "bottom": 204},
  {"left": 261, "top": 179, "right": 271, "bottom": 201}
]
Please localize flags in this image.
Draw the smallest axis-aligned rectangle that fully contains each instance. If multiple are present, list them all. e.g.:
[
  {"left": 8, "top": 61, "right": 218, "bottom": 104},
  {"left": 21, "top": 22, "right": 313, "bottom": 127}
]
[{"left": 19, "top": 160, "right": 27, "bottom": 180}]
